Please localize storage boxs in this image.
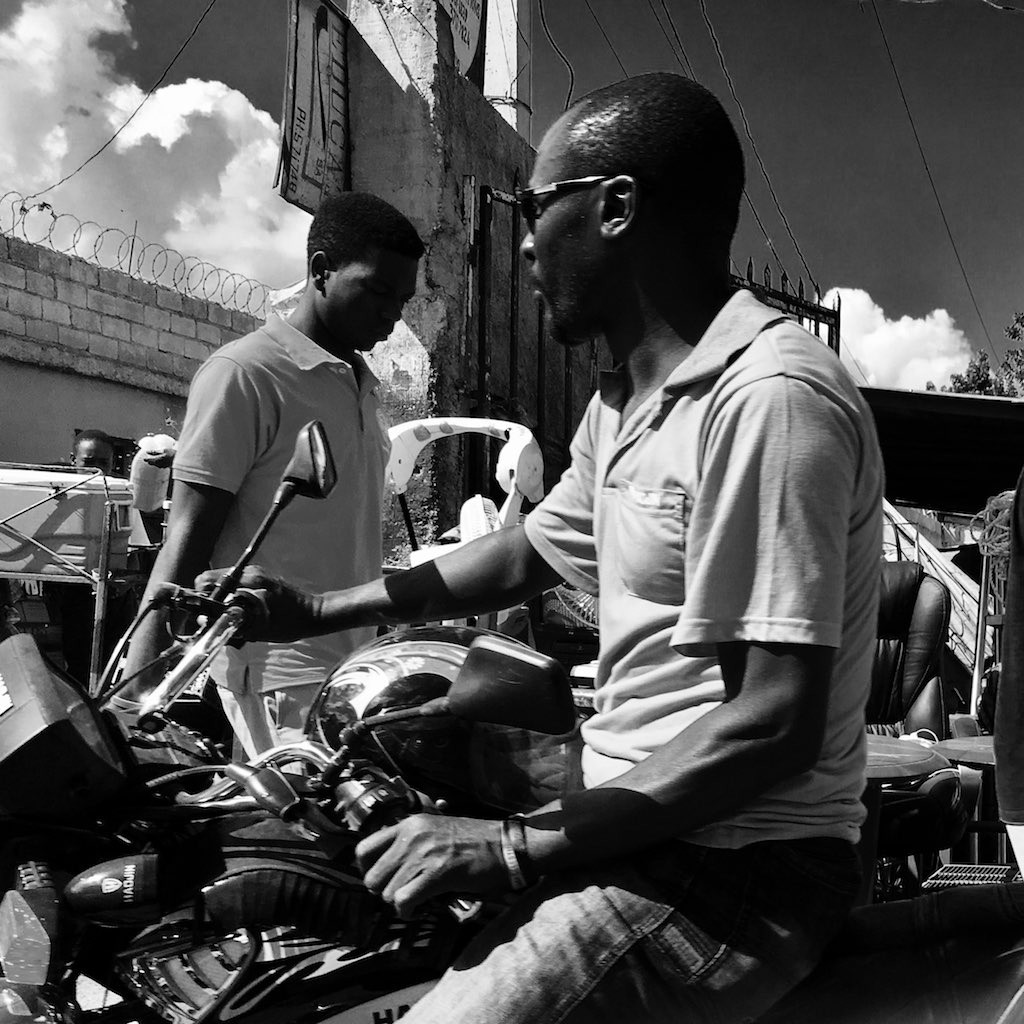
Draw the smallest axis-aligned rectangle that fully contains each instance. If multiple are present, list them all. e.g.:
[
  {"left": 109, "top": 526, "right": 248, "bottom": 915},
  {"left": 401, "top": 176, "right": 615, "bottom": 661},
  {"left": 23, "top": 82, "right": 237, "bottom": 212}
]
[{"left": 0, "top": 469, "right": 131, "bottom": 575}]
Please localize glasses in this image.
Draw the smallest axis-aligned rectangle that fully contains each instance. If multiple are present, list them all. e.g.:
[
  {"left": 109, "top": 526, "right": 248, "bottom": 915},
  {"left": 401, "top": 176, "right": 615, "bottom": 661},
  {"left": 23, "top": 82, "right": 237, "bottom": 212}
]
[{"left": 514, "top": 171, "right": 612, "bottom": 233}]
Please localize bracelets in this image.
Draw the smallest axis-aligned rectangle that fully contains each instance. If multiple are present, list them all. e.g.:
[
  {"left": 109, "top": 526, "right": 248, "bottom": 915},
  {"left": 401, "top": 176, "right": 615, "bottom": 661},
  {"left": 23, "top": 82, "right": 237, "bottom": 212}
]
[{"left": 500, "top": 811, "right": 533, "bottom": 894}]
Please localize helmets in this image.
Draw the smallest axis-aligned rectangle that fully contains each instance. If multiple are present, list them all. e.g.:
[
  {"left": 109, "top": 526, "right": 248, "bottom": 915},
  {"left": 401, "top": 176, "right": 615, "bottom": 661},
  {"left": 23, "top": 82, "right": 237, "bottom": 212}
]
[{"left": 301, "top": 623, "right": 570, "bottom": 854}]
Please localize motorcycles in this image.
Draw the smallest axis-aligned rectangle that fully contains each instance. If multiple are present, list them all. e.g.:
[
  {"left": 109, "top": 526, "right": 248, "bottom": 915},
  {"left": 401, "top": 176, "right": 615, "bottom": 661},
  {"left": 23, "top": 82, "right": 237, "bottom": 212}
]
[{"left": 0, "top": 420, "right": 1023, "bottom": 1023}]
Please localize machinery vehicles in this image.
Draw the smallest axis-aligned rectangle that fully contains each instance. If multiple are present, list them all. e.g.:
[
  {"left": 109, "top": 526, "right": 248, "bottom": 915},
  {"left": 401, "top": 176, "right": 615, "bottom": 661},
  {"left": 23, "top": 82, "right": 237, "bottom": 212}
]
[{"left": 0, "top": 432, "right": 175, "bottom": 599}]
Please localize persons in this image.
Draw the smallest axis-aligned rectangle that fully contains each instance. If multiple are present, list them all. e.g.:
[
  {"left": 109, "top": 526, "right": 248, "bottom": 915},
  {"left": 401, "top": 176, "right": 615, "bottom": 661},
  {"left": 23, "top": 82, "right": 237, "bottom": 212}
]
[
  {"left": 70, "top": 427, "right": 151, "bottom": 664},
  {"left": 191, "top": 70, "right": 889, "bottom": 1024},
  {"left": 116, "top": 192, "right": 430, "bottom": 778}
]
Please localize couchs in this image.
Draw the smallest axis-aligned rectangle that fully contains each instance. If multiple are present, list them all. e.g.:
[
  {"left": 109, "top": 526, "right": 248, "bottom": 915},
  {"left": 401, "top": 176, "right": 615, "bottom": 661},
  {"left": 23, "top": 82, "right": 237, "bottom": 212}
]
[{"left": 865, "top": 561, "right": 982, "bottom": 739}]
